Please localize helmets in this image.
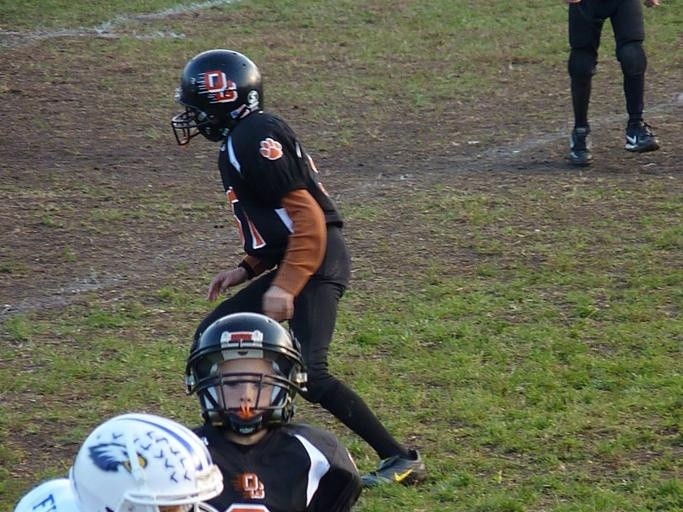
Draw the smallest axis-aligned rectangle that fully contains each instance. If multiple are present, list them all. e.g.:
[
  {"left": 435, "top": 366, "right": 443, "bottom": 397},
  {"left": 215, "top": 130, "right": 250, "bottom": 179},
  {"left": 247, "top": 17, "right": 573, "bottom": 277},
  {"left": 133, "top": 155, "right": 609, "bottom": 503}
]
[
  {"left": 186, "top": 312, "right": 308, "bottom": 449},
  {"left": 172, "top": 50, "right": 262, "bottom": 145},
  {"left": 69, "top": 414, "right": 224, "bottom": 512}
]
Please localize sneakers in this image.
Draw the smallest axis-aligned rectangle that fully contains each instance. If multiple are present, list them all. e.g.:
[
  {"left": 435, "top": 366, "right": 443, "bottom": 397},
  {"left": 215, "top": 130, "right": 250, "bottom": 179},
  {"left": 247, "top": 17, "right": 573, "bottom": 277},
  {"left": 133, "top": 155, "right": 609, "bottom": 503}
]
[
  {"left": 624, "top": 121, "right": 659, "bottom": 151},
  {"left": 359, "top": 448, "right": 427, "bottom": 487},
  {"left": 569, "top": 126, "right": 592, "bottom": 165}
]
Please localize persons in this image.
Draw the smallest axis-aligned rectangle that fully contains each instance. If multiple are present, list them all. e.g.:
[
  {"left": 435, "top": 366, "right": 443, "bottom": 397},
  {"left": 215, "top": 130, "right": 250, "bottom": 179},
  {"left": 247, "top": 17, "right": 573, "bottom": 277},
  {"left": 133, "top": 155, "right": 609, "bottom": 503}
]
[
  {"left": 168, "top": 48, "right": 430, "bottom": 489},
  {"left": 181, "top": 311, "right": 364, "bottom": 512},
  {"left": 561, "top": 0, "right": 661, "bottom": 165},
  {"left": 12, "top": 412, "right": 223, "bottom": 512}
]
